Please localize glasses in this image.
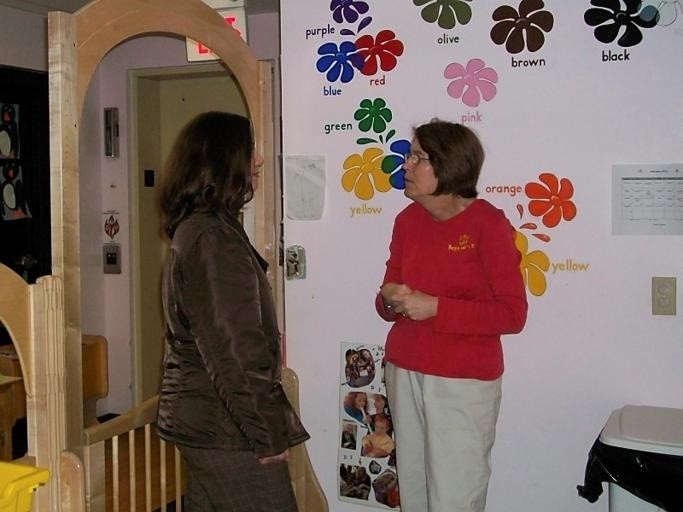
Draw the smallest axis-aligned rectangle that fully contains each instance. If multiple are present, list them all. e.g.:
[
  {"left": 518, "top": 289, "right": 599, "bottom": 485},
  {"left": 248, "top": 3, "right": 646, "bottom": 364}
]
[{"left": 404, "top": 153, "right": 429, "bottom": 166}]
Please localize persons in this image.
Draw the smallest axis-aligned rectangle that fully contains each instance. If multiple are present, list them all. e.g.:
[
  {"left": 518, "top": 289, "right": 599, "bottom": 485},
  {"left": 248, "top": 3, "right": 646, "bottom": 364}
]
[
  {"left": 154, "top": 111, "right": 310, "bottom": 512},
  {"left": 372, "top": 117, "right": 529, "bottom": 512},
  {"left": 335, "top": 347, "right": 401, "bottom": 507}
]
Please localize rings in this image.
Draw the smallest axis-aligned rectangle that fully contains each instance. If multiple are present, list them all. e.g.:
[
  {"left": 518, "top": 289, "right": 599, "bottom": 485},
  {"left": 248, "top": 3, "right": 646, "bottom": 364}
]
[{"left": 401, "top": 313, "right": 406, "bottom": 317}]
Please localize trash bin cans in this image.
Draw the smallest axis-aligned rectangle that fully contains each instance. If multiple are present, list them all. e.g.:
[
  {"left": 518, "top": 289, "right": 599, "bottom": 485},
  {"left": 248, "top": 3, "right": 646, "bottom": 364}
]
[{"left": 599, "top": 405, "right": 683, "bottom": 512}]
